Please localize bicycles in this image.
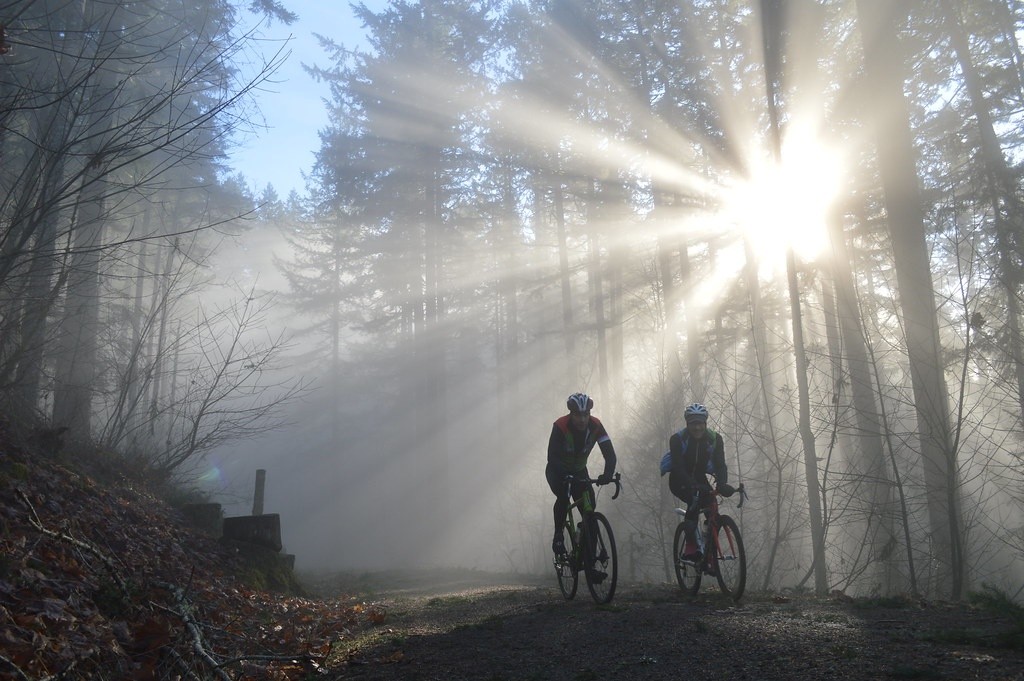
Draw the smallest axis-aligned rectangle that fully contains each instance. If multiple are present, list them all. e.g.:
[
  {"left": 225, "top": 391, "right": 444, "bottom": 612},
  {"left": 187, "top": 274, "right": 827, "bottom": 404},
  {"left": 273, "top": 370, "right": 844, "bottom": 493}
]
[
  {"left": 553, "top": 471, "right": 624, "bottom": 606},
  {"left": 672, "top": 481, "right": 749, "bottom": 602}
]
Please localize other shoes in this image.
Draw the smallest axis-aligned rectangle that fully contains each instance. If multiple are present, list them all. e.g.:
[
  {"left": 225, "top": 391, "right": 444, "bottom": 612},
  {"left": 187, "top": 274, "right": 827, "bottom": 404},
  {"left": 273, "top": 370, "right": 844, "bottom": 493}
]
[
  {"left": 684, "top": 540, "right": 697, "bottom": 558},
  {"left": 706, "top": 560, "right": 718, "bottom": 576},
  {"left": 553, "top": 537, "right": 566, "bottom": 553},
  {"left": 592, "top": 569, "right": 607, "bottom": 580}
]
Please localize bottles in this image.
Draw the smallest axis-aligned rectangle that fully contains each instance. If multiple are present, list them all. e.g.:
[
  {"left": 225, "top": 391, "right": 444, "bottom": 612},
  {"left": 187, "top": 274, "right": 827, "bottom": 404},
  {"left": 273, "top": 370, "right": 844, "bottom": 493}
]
[
  {"left": 574, "top": 522, "right": 583, "bottom": 544},
  {"left": 701, "top": 520, "right": 709, "bottom": 544}
]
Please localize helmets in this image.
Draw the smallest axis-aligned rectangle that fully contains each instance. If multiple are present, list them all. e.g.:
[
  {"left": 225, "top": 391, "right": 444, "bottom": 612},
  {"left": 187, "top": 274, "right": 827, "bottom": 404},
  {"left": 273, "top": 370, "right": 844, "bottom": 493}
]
[
  {"left": 567, "top": 393, "right": 593, "bottom": 416},
  {"left": 684, "top": 402, "right": 709, "bottom": 422}
]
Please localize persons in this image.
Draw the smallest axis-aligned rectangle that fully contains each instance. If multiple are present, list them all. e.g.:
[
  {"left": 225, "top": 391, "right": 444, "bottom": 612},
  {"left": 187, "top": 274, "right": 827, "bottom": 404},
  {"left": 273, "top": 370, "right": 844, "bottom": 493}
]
[
  {"left": 545, "top": 393, "right": 617, "bottom": 584},
  {"left": 669, "top": 403, "right": 736, "bottom": 576}
]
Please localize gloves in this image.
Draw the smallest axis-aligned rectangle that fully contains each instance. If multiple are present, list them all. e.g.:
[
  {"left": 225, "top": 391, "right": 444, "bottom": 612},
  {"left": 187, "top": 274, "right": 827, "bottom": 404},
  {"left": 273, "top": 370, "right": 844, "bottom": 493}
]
[
  {"left": 719, "top": 484, "right": 735, "bottom": 499},
  {"left": 690, "top": 484, "right": 706, "bottom": 499},
  {"left": 561, "top": 475, "right": 575, "bottom": 488},
  {"left": 597, "top": 472, "right": 612, "bottom": 486}
]
